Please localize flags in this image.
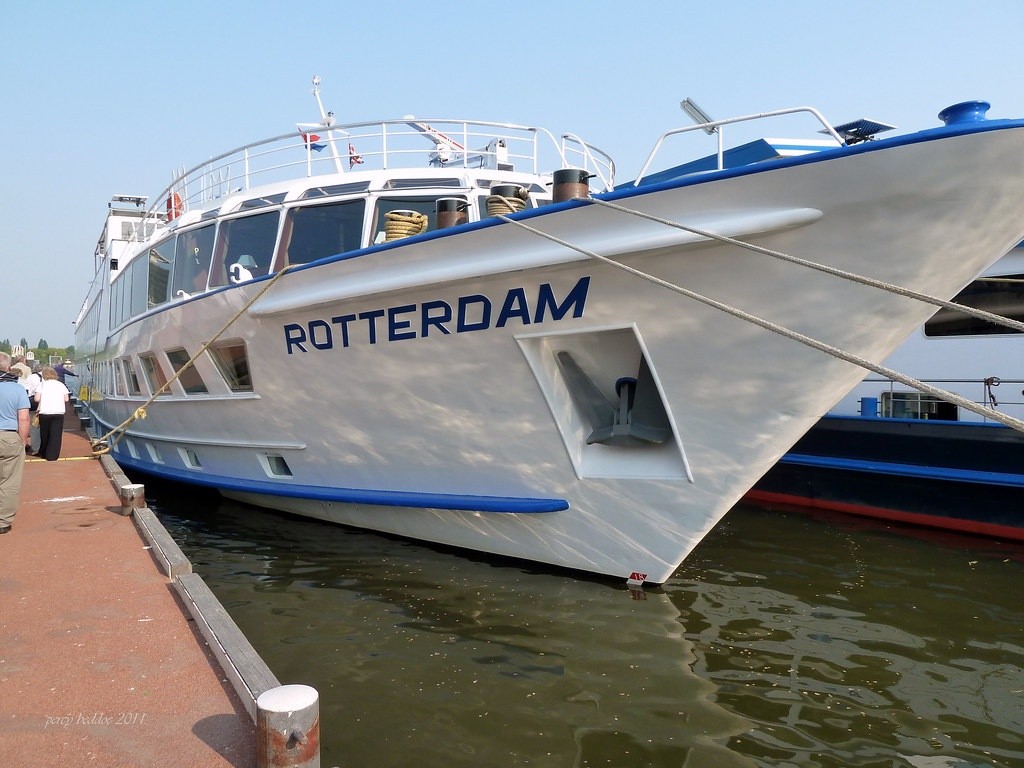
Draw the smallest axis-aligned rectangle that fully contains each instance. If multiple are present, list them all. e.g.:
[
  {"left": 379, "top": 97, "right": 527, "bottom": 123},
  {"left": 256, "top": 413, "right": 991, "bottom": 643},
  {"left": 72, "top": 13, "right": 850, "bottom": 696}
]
[
  {"left": 298, "top": 128, "right": 328, "bottom": 152},
  {"left": 349, "top": 143, "right": 364, "bottom": 170}
]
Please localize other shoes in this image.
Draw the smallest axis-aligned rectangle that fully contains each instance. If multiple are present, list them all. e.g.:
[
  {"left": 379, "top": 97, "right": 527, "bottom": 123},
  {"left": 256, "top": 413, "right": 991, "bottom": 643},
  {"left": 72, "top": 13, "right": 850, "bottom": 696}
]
[
  {"left": 0, "top": 524, "right": 11, "bottom": 534},
  {"left": 31, "top": 451, "right": 38, "bottom": 455}
]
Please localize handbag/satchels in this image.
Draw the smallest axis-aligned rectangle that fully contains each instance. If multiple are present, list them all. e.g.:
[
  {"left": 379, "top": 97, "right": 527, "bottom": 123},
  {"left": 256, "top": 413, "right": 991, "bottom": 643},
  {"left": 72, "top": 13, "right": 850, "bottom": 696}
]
[
  {"left": 28, "top": 395, "right": 39, "bottom": 411},
  {"left": 31, "top": 409, "right": 40, "bottom": 428}
]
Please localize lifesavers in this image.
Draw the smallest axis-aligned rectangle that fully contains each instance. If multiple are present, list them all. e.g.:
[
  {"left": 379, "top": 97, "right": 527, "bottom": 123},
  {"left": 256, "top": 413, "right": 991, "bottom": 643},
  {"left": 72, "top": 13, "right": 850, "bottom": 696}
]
[{"left": 166, "top": 193, "right": 181, "bottom": 222}]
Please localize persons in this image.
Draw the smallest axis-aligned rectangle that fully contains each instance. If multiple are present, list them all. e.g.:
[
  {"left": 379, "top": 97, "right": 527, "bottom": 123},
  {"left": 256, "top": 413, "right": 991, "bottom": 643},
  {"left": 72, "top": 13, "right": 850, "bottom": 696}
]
[{"left": 0, "top": 352, "right": 80, "bottom": 534}]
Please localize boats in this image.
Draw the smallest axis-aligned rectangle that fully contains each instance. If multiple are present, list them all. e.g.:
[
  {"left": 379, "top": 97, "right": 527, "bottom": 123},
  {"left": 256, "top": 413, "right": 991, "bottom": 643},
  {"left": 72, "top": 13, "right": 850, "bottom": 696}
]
[{"left": 71, "top": 74, "right": 1023, "bottom": 585}]
